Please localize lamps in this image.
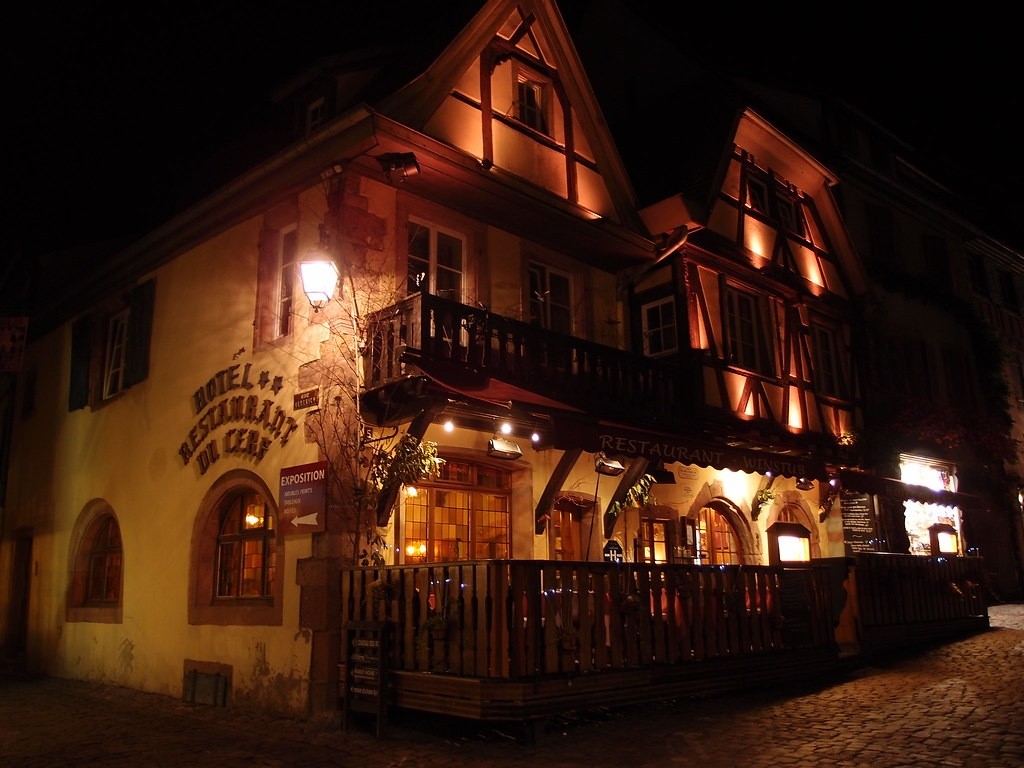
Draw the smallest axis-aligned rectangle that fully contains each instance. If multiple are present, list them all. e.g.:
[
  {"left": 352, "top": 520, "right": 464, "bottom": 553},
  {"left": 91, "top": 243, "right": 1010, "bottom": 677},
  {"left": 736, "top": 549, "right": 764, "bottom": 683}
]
[
  {"left": 482, "top": 423, "right": 523, "bottom": 461},
  {"left": 297, "top": 224, "right": 347, "bottom": 311},
  {"left": 594, "top": 451, "right": 627, "bottom": 477},
  {"left": 647, "top": 460, "right": 678, "bottom": 485},
  {"left": 792, "top": 478, "right": 815, "bottom": 491}
]
